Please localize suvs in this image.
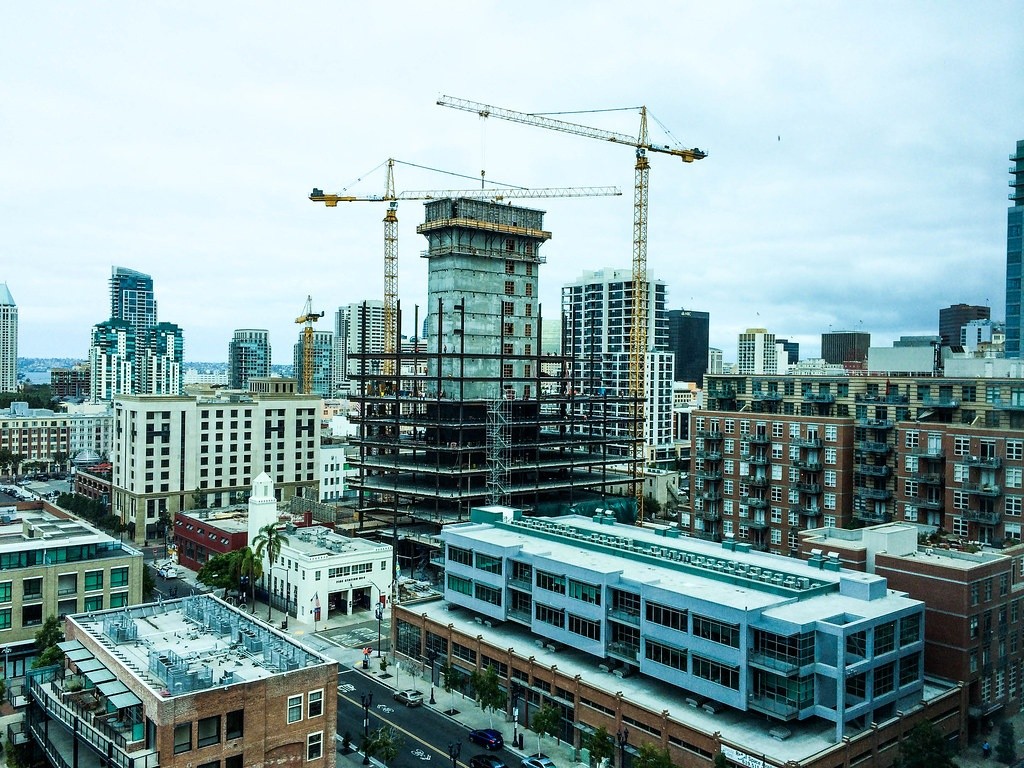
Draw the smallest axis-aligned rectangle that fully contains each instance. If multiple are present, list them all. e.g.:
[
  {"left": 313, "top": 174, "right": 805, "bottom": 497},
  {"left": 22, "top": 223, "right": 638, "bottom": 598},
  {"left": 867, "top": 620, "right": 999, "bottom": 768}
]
[
  {"left": 159, "top": 565, "right": 178, "bottom": 580},
  {"left": 393, "top": 688, "right": 423, "bottom": 708},
  {"left": 468, "top": 729, "right": 503, "bottom": 752}
]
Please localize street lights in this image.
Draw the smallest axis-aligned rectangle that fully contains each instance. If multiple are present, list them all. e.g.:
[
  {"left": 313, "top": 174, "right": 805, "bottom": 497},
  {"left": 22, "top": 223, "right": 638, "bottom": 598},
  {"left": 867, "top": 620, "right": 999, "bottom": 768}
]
[
  {"left": 426, "top": 648, "right": 438, "bottom": 705},
  {"left": 364, "top": 577, "right": 381, "bottom": 659},
  {"left": 267, "top": 566, "right": 289, "bottom": 630},
  {"left": 360, "top": 690, "right": 374, "bottom": 765},
  {"left": 510, "top": 684, "right": 520, "bottom": 748},
  {"left": 447, "top": 739, "right": 462, "bottom": 768}
]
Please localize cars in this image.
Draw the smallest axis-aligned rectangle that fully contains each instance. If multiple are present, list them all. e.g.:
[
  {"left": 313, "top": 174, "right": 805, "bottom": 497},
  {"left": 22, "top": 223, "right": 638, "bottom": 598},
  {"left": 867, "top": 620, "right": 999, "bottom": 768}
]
[
  {"left": 521, "top": 754, "right": 557, "bottom": 768},
  {"left": 17, "top": 479, "right": 32, "bottom": 486},
  {"left": 469, "top": 753, "right": 509, "bottom": 768},
  {"left": 0, "top": 482, "right": 34, "bottom": 502}
]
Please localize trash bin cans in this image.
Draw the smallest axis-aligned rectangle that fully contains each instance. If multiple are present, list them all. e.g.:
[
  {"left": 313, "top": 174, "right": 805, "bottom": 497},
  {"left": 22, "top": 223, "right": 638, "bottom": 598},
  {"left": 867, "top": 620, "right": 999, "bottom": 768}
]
[
  {"left": 282, "top": 621, "right": 286, "bottom": 629},
  {"left": 362, "top": 659, "right": 368, "bottom": 669},
  {"left": 518, "top": 733, "right": 524, "bottom": 751}
]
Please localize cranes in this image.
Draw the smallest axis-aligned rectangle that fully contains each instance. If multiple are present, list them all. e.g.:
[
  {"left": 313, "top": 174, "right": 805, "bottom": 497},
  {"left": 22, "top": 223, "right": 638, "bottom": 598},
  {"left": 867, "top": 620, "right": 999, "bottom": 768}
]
[
  {"left": 435, "top": 96, "right": 710, "bottom": 525},
  {"left": 308, "top": 158, "right": 623, "bottom": 401},
  {"left": 294, "top": 295, "right": 324, "bottom": 397}
]
